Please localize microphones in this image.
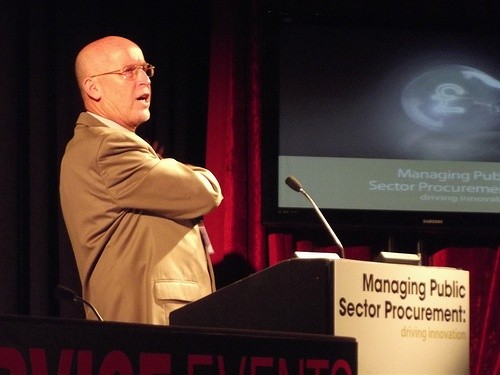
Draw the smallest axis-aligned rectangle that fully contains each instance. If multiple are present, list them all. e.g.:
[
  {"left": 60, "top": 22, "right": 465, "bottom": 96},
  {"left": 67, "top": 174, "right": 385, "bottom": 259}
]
[
  {"left": 284, "top": 176, "right": 345, "bottom": 258},
  {"left": 56, "top": 286, "right": 102, "bottom": 320}
]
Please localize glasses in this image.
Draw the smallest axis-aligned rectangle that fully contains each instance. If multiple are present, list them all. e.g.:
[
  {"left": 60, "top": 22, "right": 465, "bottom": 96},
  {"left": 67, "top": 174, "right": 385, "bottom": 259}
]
[{"left": 91, "top": 64, "right": 155, "bottom": 78}]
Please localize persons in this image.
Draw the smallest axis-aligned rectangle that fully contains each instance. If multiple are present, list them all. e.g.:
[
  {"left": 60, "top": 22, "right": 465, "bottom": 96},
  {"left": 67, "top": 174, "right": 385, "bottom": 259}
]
[{"left": 58, "top": 36, "right": 223, "bottom": 325}]
